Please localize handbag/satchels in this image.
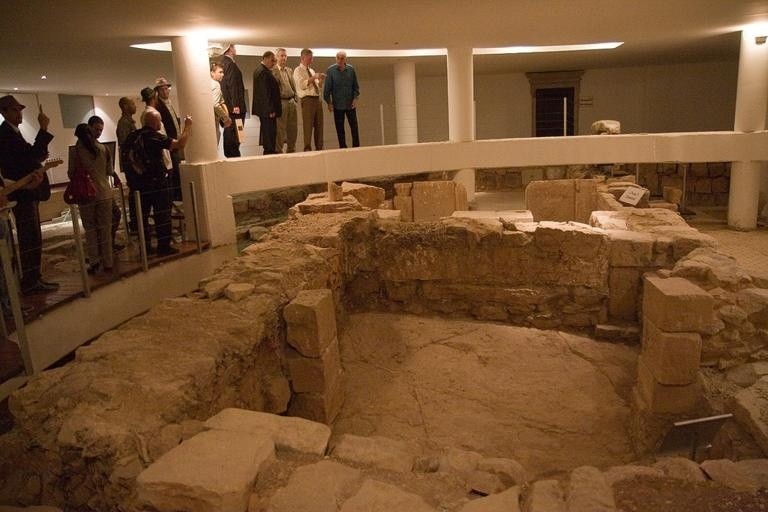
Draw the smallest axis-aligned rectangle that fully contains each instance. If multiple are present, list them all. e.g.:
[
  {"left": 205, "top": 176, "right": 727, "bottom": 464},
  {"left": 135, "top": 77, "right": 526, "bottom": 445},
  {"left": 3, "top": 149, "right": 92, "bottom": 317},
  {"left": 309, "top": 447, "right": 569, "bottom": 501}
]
[{"left": 64, "top": 172, "right": 96, "bottom": 204}]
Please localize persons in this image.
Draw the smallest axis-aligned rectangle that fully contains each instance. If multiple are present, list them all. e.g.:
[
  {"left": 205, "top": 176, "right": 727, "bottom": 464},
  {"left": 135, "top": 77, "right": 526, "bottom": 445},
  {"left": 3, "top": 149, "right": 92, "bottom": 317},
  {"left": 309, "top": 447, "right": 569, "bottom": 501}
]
[
  {"left": 219, "top": 41, "right": 247, "bottom": 158},
  {"left": 323, "top": 52, "right": 360, "bottom": 148},
  {"left": 252, "top": 52, "right": 282, "bottom": 154},
  {"left": 0, "top": 94, "right": 60, "bottom": 296},
  {"left": 0, "top": 167, "right": 45, "bottom": 319},
  {"left": 293, "top": 49, "right": 326, "bottom": 152},
  {"left": 210, "top": 56, "right": 233, "bottom": 146},
  {"left": 270, "top": 49, "right": 299, "bottom": 154},
  {"left": 67, "top": 78, "right": 194, "bottom": 276}
]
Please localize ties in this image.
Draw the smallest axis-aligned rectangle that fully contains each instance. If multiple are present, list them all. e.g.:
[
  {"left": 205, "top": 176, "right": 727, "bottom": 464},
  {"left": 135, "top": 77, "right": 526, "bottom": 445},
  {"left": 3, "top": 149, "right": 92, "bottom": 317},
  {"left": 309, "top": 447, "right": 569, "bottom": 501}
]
[{"left": 307, "top": 69, "right": 319, "bottom": 94}]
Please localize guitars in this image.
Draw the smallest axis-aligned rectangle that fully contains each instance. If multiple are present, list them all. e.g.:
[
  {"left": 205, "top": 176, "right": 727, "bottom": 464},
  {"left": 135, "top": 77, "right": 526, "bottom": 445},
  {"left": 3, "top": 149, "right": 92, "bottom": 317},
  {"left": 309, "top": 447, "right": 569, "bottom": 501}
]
[{"left": 0, "top": 159, "right": 63, "bottom": 227}]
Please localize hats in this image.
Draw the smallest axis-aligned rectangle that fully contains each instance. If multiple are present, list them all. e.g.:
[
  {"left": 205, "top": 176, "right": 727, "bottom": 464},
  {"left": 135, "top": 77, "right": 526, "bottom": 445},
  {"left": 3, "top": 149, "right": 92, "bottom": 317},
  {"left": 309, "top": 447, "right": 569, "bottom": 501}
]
[
  {"left": 0, "top": 96, "right": 25, "bottom": 113},
  {"left": 220, "top": 43, "right": 231, "bottom": 55},
  {"left": 141, "top": 78, "right": 171, "bottom": 101}
]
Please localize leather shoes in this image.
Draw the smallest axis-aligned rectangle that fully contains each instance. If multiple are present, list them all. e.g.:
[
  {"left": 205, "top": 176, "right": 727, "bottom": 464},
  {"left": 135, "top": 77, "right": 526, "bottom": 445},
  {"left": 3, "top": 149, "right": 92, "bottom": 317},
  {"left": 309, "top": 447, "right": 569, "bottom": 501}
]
[{"left": 22, "top": 279, "right": 58, "bottom": 295}]
